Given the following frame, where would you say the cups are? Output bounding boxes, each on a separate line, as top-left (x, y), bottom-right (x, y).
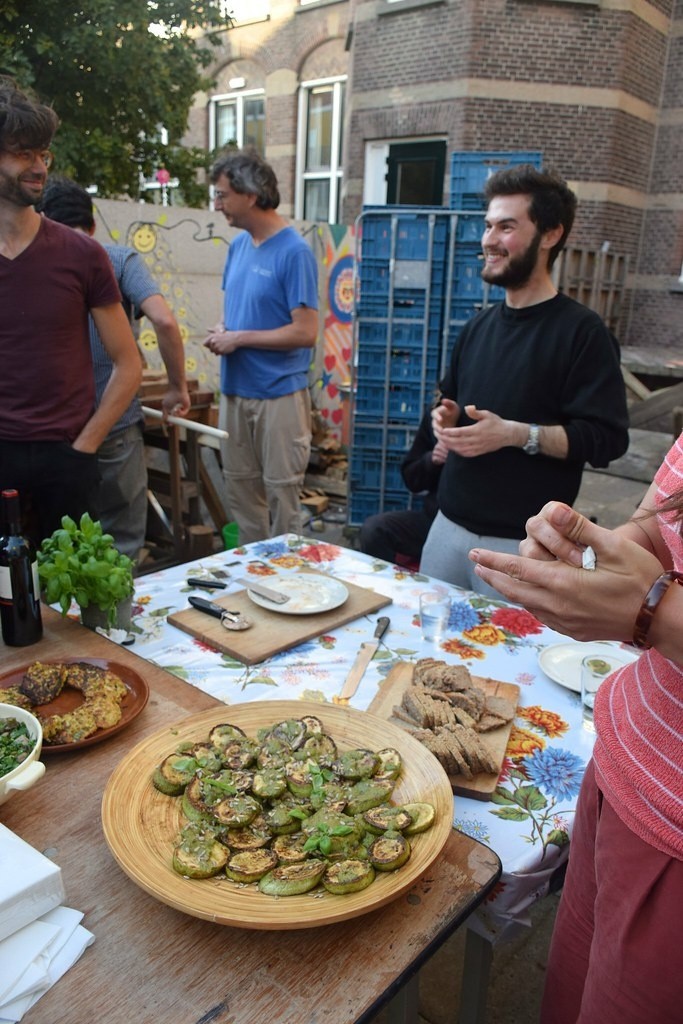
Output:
top-left (579, 653), bottom-right (627, 731)
top-left (418, 592), bottom-right (452, 642)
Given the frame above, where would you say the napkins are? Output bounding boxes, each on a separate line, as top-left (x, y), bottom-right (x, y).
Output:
top-left (0, 822), bottom-right (96, 1024)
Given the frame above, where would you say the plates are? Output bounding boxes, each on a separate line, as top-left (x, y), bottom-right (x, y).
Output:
top-left (0, 655), bottom-right (150, 751)
top-left (246, 571), bottom-right (349, 616)
top-left (537, 642), bottom-right (643, 696)
top-left (362, 661), bottom-right (520, 801)
top-left (100, 700), bottom-right (454, 930)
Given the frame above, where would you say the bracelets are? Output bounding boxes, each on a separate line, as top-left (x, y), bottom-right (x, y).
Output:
top-left (632, 569), bottom-right (683, 650)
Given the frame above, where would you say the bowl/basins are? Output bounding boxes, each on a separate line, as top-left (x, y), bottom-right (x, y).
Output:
top-left (0, 702), bottom-right (45, 812)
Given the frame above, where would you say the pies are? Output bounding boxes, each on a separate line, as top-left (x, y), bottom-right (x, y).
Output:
top-left (0, 660), bottom-right (126, 744)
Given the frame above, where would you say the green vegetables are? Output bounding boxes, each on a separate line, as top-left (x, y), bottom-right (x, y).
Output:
top-left (173, 727), bottom-right (352, 854)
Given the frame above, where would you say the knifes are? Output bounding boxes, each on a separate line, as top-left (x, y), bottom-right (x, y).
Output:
top-left (338, 616), bottom-right (390, 699)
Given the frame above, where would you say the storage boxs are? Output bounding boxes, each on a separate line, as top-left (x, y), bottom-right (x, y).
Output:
top-left (348, 152), bottom-right (542, 523)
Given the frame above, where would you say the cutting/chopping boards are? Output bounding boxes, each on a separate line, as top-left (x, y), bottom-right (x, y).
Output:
top-left (166, 566), bottom-right (393, 665)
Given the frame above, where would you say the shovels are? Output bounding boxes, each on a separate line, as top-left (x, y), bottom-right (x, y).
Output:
top-left (187, 578), bottom-right (290, 604)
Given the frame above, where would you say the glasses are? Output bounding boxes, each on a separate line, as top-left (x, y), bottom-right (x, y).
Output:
top-left (4, 150), bottom-right (53, 169)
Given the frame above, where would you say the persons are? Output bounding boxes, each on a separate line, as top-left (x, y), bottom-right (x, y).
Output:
top-left (418, 165), bottom-right (630, 608)
top-left (0, 72), bottom-right (144, 544)
top-left (467, 433), bottom-right (683, 1024)
top-left (32, 180), bottom-right (192, 579)
top-left (361, 388), bottom-right (448, 566)
top-left (204, 152), bottom-right (320, 544)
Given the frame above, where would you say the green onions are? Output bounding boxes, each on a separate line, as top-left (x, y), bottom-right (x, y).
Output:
top-left (0, 721), bottom-right (37, 776)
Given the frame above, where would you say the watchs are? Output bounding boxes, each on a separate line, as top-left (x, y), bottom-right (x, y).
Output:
top-left (522, 423), bottom-right (541, 455)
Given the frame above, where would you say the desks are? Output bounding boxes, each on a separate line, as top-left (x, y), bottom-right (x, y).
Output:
top-left (51, 532), bottom-right (645, 1024)
top-left (0, 603), bottom-right (501, 1024)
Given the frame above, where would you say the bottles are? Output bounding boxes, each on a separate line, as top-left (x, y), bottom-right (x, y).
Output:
top-left (0, 489), bottom-right (42, 648)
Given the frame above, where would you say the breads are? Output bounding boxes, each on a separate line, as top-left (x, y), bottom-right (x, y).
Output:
top-left (390, 658), bottom-right (516, 779)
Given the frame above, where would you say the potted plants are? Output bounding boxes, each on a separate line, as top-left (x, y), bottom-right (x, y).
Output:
top-left (36, 512), bottom-right (138, 631)
